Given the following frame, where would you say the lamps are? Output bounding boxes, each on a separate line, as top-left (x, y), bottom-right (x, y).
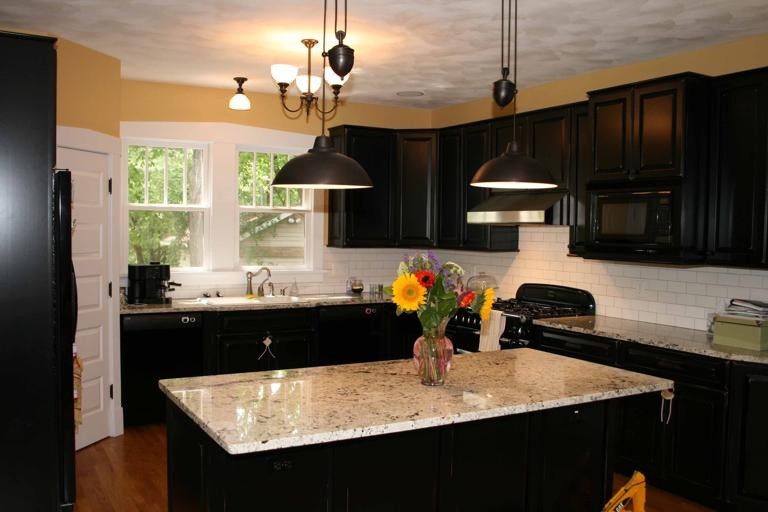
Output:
top-left (271, 40), bottom-right (351, 121)
top-left (229, 77), bottom-right (250, 111)
top-left (469, 1), bottom-right (558, 188)
top-left (271, 0), bottom-right (375, 189)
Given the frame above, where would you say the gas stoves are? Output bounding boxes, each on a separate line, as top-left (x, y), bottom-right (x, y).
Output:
top-left (492, 298), bottom-right (577, 320)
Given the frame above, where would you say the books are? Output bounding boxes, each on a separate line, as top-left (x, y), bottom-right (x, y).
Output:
top-left (725, 299), bottom-right (768, 317)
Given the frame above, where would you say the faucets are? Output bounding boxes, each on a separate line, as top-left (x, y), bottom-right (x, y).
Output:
top-left (245, 266), bottom-right (271, 296)
top-left (267, 282), bottom-right (276, 296)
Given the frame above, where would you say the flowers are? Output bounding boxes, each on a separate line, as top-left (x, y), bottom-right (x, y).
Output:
top-left (383, 251), bottom-right (494, 387)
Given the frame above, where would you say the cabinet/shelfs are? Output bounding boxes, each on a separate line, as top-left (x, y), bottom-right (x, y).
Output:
top-left (715, 67), bottom-right (768, 269)
top-left (588, 71), bottom-right (712, 182)
top-left (220, 308), bottom-right (317, 373)
top-left (308, 300), bottom-right (399, 368)
top-left (540, 327), bottom-right (617, 367)
top-left (396, 129), bottom-right (437, 249)
top-left (324, 124), bottom-right (396, 248)
top-left (618, 340), bottom-right (730, 512)
top-left (724, 362), bottom-right (768, 512)
top-left (491, 105), bottom-right (571, 251)
top-left (122, 311), bottom-right (212, 426)
top-left (439, 119), bottom-right (490, 253)
top-left (571, 100), bottom-right (594, 249)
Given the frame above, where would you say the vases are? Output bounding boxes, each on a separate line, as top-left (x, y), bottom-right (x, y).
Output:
top-left (412, 318), bottom-right (454, 386)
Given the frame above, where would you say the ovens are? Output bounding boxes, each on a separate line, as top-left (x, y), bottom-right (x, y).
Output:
top-left (455, 327), bottom-right (528, 356)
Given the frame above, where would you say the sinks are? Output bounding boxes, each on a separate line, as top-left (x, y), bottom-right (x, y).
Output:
top-left (201, 296), bottom-right (259, 306)
top-left (256, 296), bottom-right (298, 304)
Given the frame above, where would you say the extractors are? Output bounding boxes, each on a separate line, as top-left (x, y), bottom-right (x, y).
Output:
top-left (467, 192), bottom-right (570, 227)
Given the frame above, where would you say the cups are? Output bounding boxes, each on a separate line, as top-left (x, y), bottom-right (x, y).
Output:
top-left (370, 284), bottom-right (384, 293)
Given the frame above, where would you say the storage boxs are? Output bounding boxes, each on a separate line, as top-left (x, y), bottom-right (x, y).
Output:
top-left (712, 315), bottom-right (768, 351)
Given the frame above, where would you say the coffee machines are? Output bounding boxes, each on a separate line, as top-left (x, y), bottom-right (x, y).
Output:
top-left (127, 259), bottom-right (182, 304)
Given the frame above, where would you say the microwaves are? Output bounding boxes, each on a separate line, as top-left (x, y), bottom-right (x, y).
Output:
top-left (593, 191), bottom-right (672, 244)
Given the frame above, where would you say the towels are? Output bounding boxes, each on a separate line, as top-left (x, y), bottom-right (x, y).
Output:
top-left (477, 309), bottom-right (506, 352)
